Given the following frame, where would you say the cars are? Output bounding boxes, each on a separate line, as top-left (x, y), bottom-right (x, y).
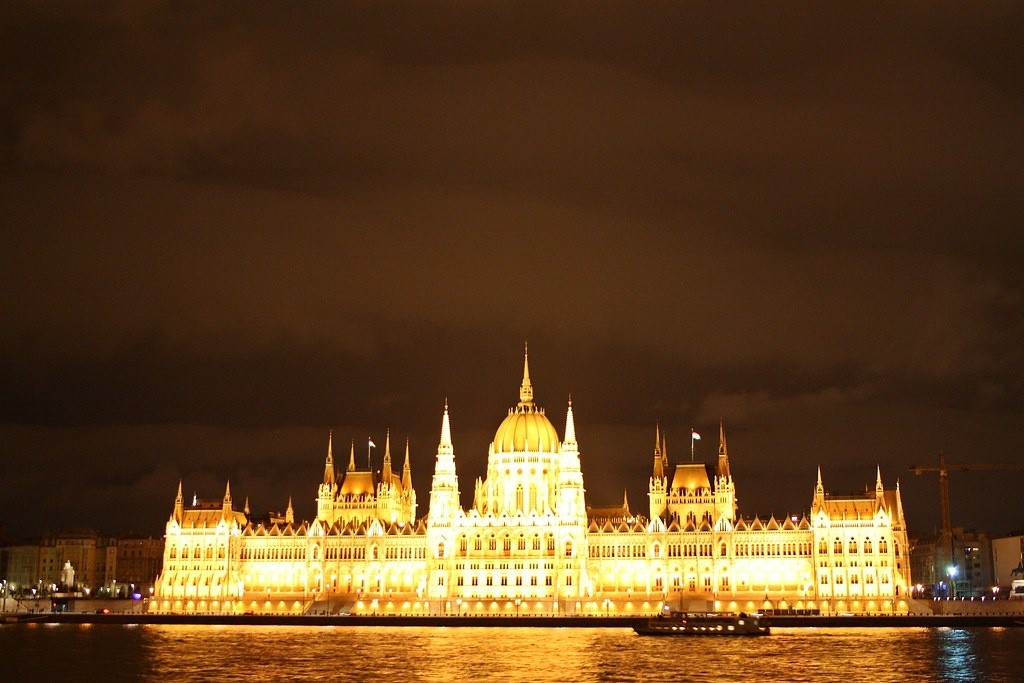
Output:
top-left (95, 609), bottom-right (109, 614)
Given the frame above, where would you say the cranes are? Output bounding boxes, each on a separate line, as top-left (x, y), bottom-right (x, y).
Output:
top-left (907, 450), bottom-right (1024, 577)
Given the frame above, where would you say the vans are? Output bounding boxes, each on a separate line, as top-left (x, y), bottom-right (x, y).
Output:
top-left (1008, 579), bottom-right (1024, 599)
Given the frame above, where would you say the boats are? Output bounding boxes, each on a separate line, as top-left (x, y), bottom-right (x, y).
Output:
top-left (627, 612), bottom-right (771, 636)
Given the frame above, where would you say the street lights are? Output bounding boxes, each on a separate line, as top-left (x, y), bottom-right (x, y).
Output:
top-left (605, 599), bottom-right (610, 617)
top-left (948, 567), bottom-right (957, 599)
top-left (514, 599), bottom-right (520, 617)
top-left (457, 599), bottom-right (462, 616)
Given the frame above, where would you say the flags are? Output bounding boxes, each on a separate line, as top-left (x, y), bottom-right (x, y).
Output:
top-left (369, 440), bottom-right (376, 447)
top-left (692, 432), bottom-right (701, 440)
